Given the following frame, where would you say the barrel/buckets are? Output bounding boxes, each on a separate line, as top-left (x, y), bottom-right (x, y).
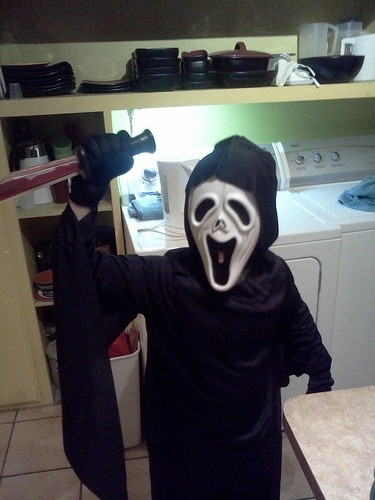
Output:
top-left (45, 340), bottom-right (62, 399)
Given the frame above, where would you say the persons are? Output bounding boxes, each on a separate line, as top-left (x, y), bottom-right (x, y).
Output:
top-left (50, 129), bottom-right (335, 500)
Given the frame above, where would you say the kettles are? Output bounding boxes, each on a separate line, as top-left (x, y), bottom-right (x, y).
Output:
top-left (156, 154), bottom-right (203, 234)
top-left (8, 136), bottom-right (56, 204)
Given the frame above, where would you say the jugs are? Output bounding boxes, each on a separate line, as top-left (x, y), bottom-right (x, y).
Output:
top-left (295, 22), bottom-right (337, 58)
top-left (339, 32), bottom-right (375, 82)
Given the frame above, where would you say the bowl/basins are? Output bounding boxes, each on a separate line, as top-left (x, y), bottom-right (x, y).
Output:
top-left (132, 40), bottom-right (278, 91)
top-left (297, 54), bottom-right (366, 85)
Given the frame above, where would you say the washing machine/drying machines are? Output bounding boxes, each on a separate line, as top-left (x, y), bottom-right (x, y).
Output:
top-left (254, 133), bottom-right (374, 401)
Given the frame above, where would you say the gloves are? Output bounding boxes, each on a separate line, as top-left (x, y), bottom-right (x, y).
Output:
top-left (70, 130), bottom-right (135, 206)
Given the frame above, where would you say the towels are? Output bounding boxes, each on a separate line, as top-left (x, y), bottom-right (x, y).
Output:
top-left (339, 175), bottom-right (375, 211)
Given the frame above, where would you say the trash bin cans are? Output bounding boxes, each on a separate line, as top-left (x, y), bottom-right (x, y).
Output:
top-left (109, 340), bottom-right (141, 449)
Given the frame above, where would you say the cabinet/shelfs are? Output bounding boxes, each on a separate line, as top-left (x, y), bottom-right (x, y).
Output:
top-left (0, 0), bottom-right (375, 412)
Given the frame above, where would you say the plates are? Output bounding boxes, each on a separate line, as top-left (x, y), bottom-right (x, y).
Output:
top-left (33, 269), bottom-right (54, 303)
top-left (80, 79), bottom-right (132, 93)
top-left (0, 60), bottom-right (77, 98)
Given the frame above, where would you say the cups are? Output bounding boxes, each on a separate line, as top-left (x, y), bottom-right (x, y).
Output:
top-left (335, 21), bottom-right (366, 54)
top-left (18, 191), bottom-right (34, 211)
top-left (50, 179), bottom-right (70, 203)
top-left (8, 83), bottom-right (24, 99)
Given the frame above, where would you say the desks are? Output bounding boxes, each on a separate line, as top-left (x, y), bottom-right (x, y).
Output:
top-left (283, 386), bottom-right (375, 500)
